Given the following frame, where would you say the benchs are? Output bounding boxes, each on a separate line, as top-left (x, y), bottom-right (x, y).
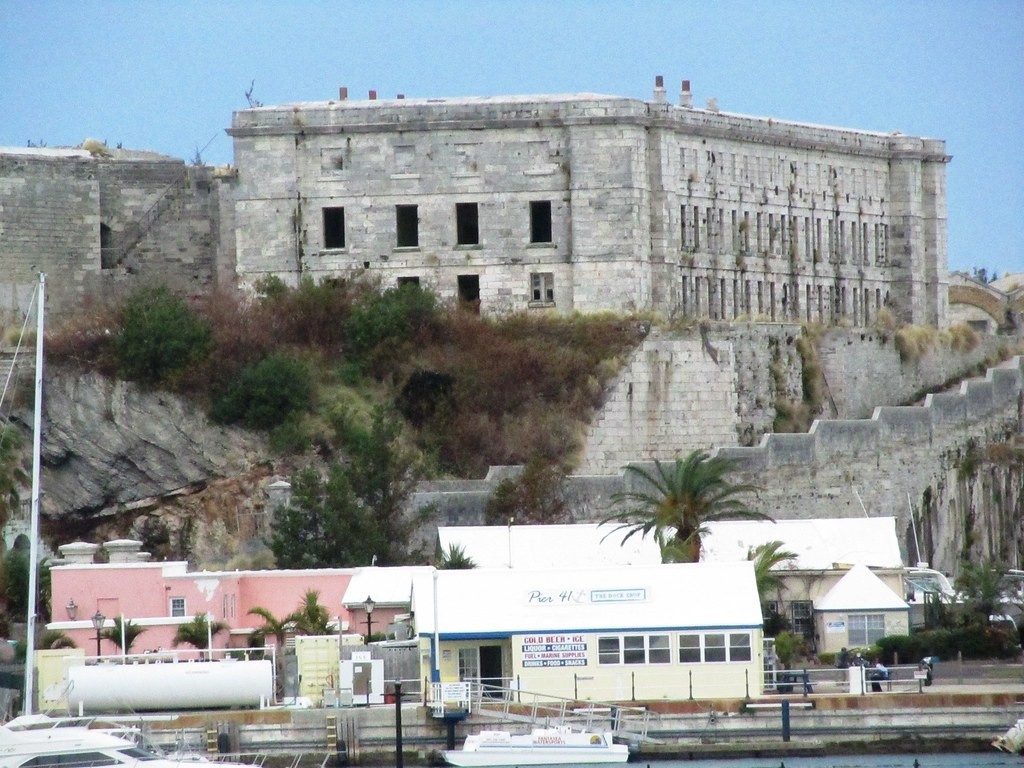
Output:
top-left (865, 671), bottom-right (892, 692)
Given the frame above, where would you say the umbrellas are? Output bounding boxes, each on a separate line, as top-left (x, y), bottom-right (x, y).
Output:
top-left (920, 657), bottom-right (938, 663)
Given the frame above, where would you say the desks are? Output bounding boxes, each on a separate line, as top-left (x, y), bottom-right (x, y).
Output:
top-left (782, 674), bottom-right (813, 693)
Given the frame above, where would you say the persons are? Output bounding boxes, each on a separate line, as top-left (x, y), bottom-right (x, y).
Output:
top-left (922, 663), bottom-right (934, 686)
top-left (871, 658), bottom-right (888, 692)
top-left (835, 647), bottom-right (850, 686)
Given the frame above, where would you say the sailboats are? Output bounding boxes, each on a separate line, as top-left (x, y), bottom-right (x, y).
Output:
top-left (0, 270), bottom-right (351, 768)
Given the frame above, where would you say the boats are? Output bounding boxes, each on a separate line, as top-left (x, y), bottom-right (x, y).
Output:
top-left (852, 488), bottom-right (1024, 648)
top-left (437, 715), bottom-right (633, 768)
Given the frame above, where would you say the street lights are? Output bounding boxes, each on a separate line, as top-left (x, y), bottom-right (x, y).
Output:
top-left (88, 610), bottom-right (110, 656)
top-left (359, 595), bottom-right (379, 643)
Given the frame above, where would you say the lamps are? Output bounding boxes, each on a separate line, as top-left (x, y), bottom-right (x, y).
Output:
top-left (65, 598), bottom-right (78, 621)
